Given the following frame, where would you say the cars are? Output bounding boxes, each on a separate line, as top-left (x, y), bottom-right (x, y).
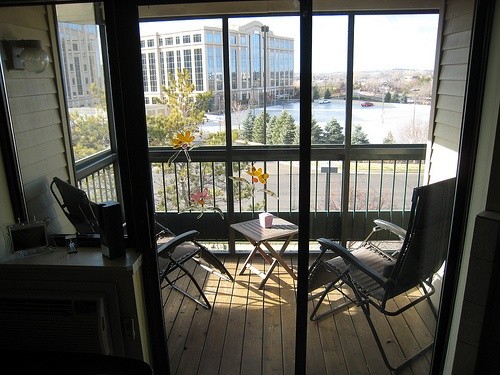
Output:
top-left (318, 98), bottom-right (331, 104)
top-left (361, 102), bottom-right (373, 107)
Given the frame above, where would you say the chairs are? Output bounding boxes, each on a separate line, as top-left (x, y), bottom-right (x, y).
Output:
top-left (49, 176), bottom-right (235, 310)
top-left (296, 175), bottom-right (457, 372)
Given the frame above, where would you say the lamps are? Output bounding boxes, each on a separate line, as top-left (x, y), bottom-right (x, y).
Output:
top-left (2, 39), bottom-right (50, 74)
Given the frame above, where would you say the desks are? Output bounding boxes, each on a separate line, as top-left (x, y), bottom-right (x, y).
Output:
top-left (229, 214), bottom-right (299, 292)
